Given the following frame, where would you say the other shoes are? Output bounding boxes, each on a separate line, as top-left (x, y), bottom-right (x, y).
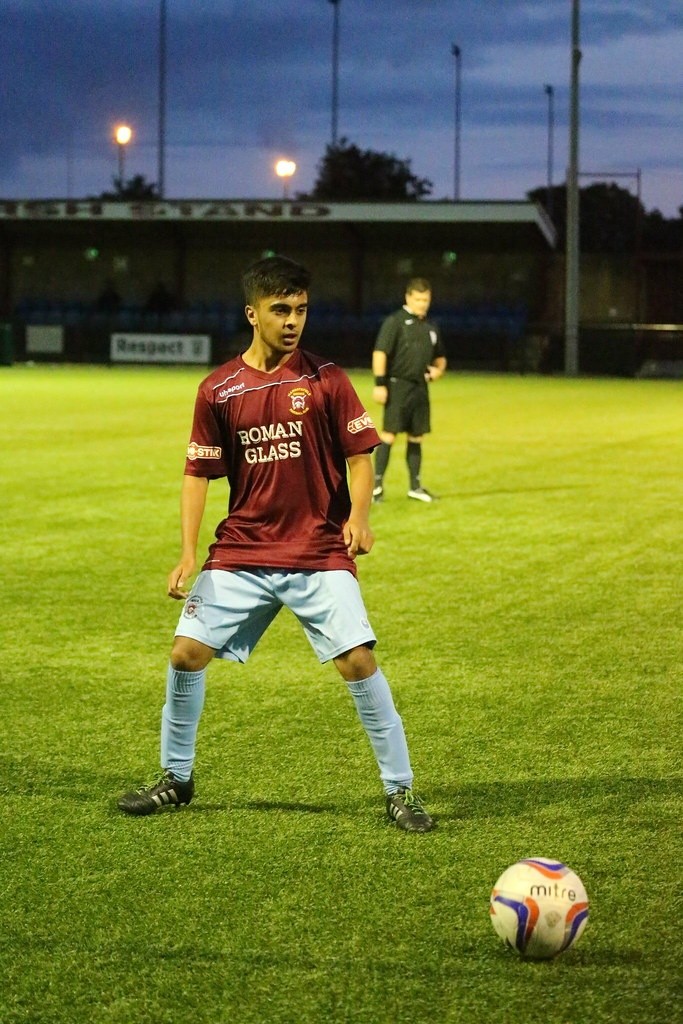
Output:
top-left (372, 485), bottom-right (384, 502)
top-left (407, 487), bottom-right (434, 503)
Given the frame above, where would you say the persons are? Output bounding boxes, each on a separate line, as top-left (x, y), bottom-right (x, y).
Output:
top-left (117, 256), bottom-right (434, 833)
top-left (97, 287), bottom-right (121, 313)
top-left (140, 283), bottom-right (174, 314)
top-left (370, 277), bottom-right (447, 502)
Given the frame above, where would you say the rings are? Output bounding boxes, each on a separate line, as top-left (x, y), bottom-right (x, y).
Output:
top-left (430, 378), bottom-right (432, 380)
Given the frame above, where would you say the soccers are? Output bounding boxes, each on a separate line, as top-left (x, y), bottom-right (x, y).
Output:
top-left (488, 856), bottom-right (590, 959)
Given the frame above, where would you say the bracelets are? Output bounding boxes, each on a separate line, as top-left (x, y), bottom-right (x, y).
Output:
top-left (375, 377), bottom-right (386, 386)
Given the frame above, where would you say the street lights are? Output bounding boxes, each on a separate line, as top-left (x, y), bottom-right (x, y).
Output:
top-left (543, 83), bottom-right (554, 187)
top-left (116, 126), bottom-right (131, 192)
top-left (449, 44), bottom-right (463, 202)
top-left (275, 159), bottom-right (298, 201)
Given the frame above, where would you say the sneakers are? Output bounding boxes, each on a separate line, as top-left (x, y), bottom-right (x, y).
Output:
top-left (385, 785), bottom-right (433, 833)
top-left (117, 771), bottom-right (195, 815)
